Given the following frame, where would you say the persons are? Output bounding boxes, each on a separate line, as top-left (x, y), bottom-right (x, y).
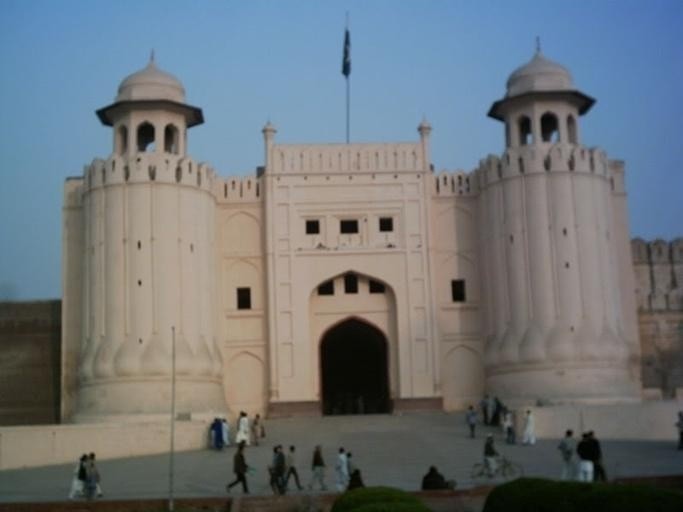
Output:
top-left (207, 411), bottom-right (262, 447)
top-left (556, 428), bottom-right (606, 483)
top-left (674, 410), bottom-right (682, 451)
top-left (226, 443), bottom-right (249, 494)
top-left (69, 453), bottom-right (103, 500)
top-left (483, 434), bottom-right (499, 479)
top-left (421, 466), bottom-right (447, 489)
top-left (268, 445), bottom-right (363, 494)
top-left (464, 394), bottom-right (536, 446)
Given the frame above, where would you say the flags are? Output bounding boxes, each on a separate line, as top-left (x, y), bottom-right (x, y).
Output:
top-left (341, 30), bottom-right (351, 76)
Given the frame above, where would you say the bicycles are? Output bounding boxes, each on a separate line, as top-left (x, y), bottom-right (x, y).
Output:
top-left (472, 449), bottom-right (523, 486)
